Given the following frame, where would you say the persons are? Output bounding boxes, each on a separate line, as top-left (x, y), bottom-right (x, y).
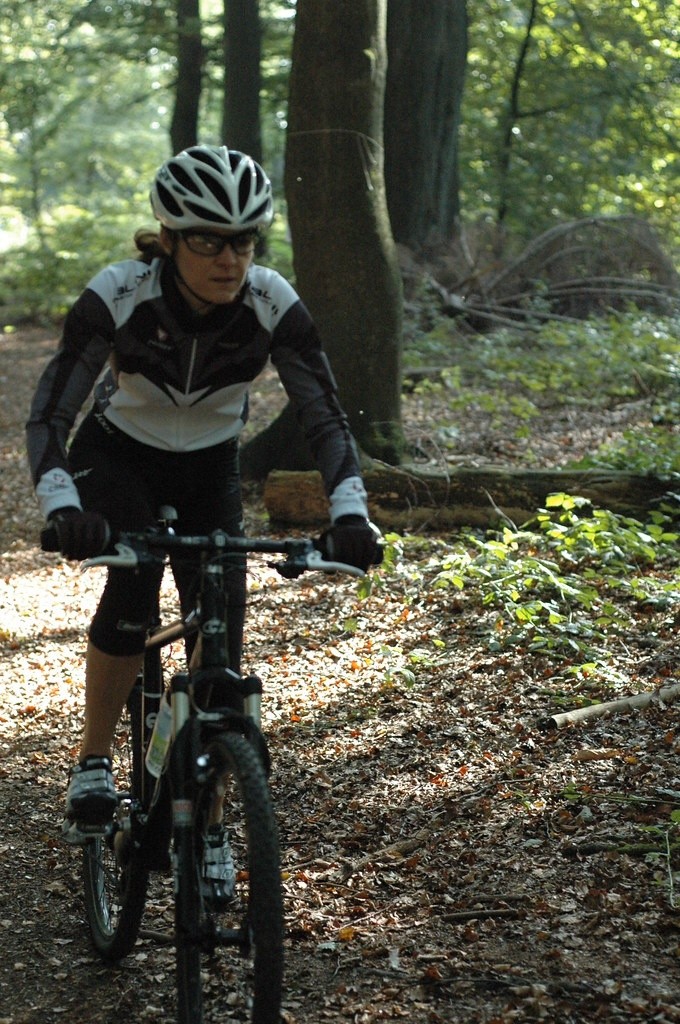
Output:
top-left (26, 144), bottom-right (383, 915)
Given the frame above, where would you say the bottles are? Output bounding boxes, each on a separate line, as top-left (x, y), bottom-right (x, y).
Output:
top-left (145, 687), bottom-right (171, 778)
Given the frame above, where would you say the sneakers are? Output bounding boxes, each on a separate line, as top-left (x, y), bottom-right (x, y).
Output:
top-left (66, 754), bottom-right (118, 821)
top-left (203, 823), bottom-right (236, 902)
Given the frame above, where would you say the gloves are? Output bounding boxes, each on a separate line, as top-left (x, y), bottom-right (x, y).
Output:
top-left (318, 515), bottom-right (383, 574)
top-left (49, 509), bottom-right (117, 561)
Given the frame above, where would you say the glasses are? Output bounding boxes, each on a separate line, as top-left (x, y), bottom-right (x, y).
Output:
top-left (180, 227), bottom-right (263, 255)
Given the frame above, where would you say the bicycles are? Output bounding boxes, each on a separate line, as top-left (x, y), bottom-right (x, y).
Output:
top-left (37, 504), bottom-right (387, 1023)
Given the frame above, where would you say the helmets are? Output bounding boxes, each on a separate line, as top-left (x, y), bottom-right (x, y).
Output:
top-left (150, 143), bottom-right (273, 233)
